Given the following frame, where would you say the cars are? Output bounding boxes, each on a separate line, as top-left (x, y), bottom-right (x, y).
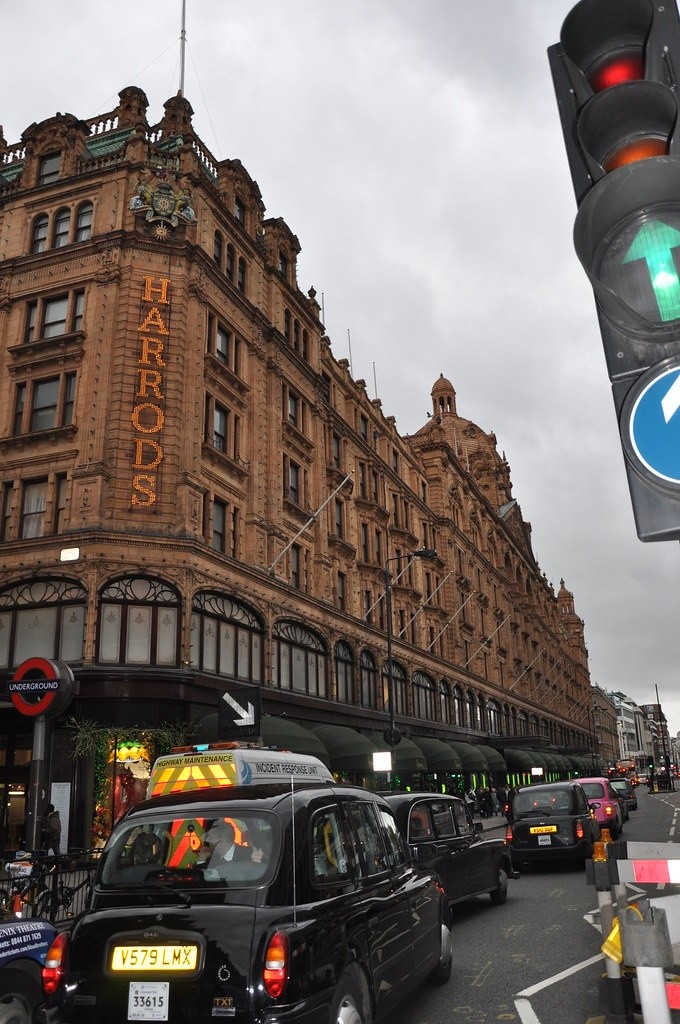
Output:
top-left (40, 741), bottom-right (453, 1024)
top-left (381, 792), bottom-right (511, 905)
top-left (571, 777), bottom-right (625, 841)
top-left (505, 780), bottom-right (602, 880)
top-left (611, 758), bottom-right (651, 824)
top-left (0, 916), bottom-right (59, 1024)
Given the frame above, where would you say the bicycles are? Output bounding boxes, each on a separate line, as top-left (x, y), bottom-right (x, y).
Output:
top-left (0, 849), bottom-right (104, 922)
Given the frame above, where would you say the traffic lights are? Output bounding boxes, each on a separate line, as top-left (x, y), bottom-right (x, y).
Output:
top-left (647, 756), bottom-right (654, 770)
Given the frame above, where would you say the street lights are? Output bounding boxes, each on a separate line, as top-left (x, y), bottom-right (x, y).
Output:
top-left (592, 707), bottom-right (609, 776)
top-left (384, 547), bottom-right (438, 791)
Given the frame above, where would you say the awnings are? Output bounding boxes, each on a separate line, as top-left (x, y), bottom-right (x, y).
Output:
top-left (261, 714), bottom-right (610, 769)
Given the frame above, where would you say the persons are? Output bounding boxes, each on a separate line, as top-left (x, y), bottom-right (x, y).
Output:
top-left (416, 784), bottom-right (516, 820)
top-left (204, 822), bottom-right (278, 869)
top-left (40, 805), bottom-right (76, 871)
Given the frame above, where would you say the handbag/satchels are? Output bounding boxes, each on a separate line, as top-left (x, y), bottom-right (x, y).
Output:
top-left (496, 805), bottom-right (501, 812)
top-left (468, 792), bottom-right (478, 802)
